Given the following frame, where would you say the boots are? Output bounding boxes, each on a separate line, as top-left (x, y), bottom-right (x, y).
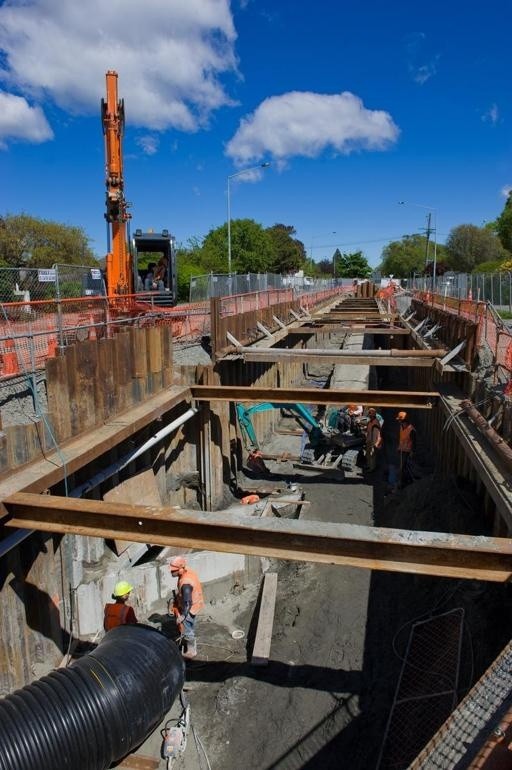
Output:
top-left (183, 639), bottom-right (196, 658)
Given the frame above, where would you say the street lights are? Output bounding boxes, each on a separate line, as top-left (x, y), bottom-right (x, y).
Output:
top-left (398, 200), bottom-right (437, 290)
top-left (310, 231), bottom-right (336, 273)
top-left (226, 162), bottom-right (270, 276)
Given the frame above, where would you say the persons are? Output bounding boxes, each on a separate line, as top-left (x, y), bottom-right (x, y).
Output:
top-left (167, 554), bottom-right (204, 659)
top-left (102, 581), bottom-right (138, 631)
top-left (143, 258), bottom-right (169, 292)
top-left (339, 405), bottom-right (419, 494)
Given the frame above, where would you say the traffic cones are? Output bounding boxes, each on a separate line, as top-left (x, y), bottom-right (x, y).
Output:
top-left (88, 313), bottom-right (97, 340)
top-left (3, 335), bottom-right (18, 374)
top-left (47, 321), bottom-right (58, 358)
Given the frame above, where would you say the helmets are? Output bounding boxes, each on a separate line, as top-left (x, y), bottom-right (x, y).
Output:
top-left (396, 412), bottom-right (408, 419)
top-left (368, 408), bottom-right (376, 416)
top-left (170, 557), bottom-right (186, 570)
top-left (114, 581), bottom-right (134, 597)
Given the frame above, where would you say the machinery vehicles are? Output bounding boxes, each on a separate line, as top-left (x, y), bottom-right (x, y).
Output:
top-left (103, 68), bottom-right (182, 314)
top-left (234, 402), bottom-right (386, 478)
